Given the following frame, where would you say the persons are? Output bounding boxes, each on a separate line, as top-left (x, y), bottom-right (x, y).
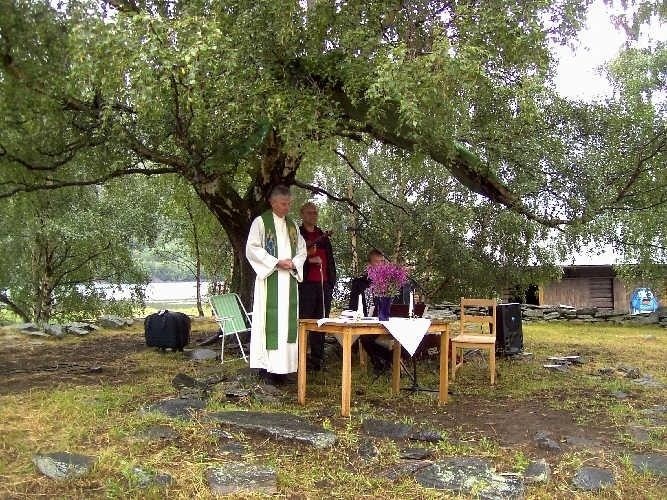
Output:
top-left (245, 186), bottom-right (308, 379)
top-left (298, 202), bottom-right (337, 371)
top-left (348, 248), bottom-right (394, 374)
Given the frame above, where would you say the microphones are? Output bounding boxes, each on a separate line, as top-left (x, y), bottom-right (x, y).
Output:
top-left (345, 226), bottom-right (364, 232)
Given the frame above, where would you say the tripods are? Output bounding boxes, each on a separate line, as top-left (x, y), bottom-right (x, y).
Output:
top-left (400, 290), bottom-right (452, 398)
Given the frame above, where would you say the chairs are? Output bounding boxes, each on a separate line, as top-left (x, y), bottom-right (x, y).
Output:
top-left (206, 291), bottom-right (252, 365)
top-left (450, 295), bottom-right (499, 386)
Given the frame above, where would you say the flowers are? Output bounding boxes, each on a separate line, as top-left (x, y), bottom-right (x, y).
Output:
top-left (365, 261), bottom-right (410, 300)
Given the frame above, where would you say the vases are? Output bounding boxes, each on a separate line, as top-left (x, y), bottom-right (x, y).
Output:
top-left (372, 296), bottom-right (394, 321)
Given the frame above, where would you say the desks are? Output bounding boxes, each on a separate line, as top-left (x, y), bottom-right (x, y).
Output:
top-left (294, 317), bottom-right (454, 418)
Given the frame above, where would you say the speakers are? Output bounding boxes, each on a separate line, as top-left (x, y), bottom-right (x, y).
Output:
top-left (489, 302), bottom-right (522, 355)
